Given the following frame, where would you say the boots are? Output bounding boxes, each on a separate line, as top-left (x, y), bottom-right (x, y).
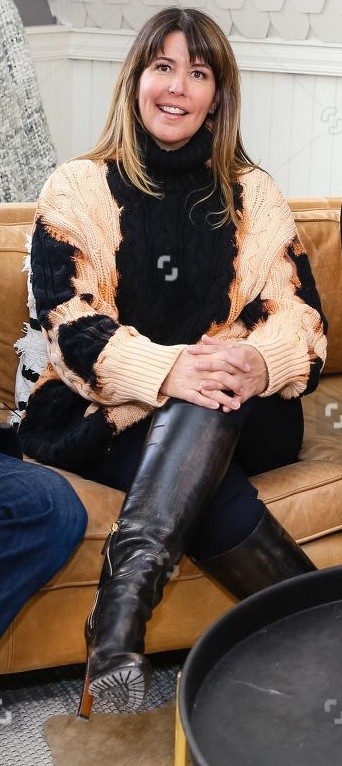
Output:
top-left (77, 399), bottom-right (243, 718)
top-left (190, 498), bottom-right (318, 602)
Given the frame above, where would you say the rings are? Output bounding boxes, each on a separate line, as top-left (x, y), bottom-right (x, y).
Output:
top-left (199, 380), bottom-right (205, 390)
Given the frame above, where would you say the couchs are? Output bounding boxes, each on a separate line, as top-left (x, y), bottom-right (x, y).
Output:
top-left (0, 195), bottom-right (342, 679)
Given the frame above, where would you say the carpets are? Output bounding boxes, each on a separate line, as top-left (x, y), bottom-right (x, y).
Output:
top-left (43, 697), bottom-right (175, 766)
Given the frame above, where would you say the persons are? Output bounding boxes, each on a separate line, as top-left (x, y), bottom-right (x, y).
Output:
top-left (12, 6), bottom-right (328, 717)
top-left (0, 423), bottom-right (90, 703)
